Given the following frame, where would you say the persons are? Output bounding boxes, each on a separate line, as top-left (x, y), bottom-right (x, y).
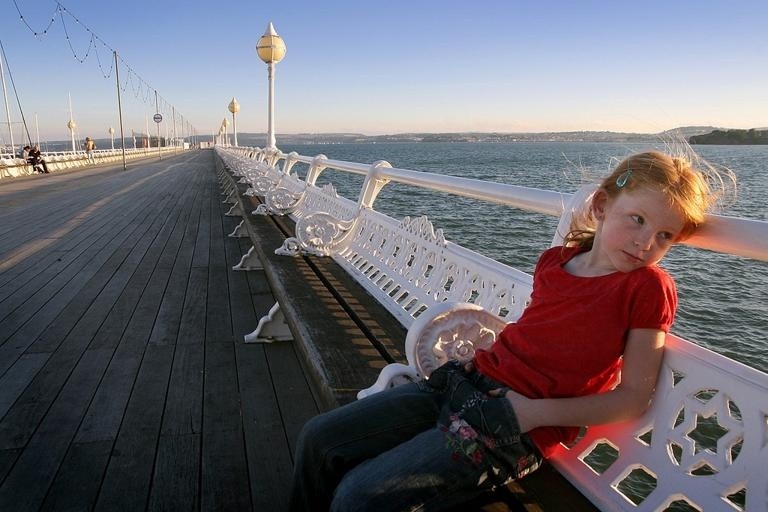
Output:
top-left (84, 137), bottom-right (96, 163)
top-left (287, 151), bottom-right (710, 512)
top-left (21, 146), bottom-right (39, 174)
top-left (28, 144), bottom-right (50, 174)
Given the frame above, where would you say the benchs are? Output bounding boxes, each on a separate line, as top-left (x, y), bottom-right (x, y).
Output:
top-left (213, 146), bottom-right (767, 512)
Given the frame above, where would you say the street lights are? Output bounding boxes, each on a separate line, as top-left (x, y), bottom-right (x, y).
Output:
top-left (216, 119), bottom-right (228, 145)
top-left (67, 120), bottom-right (78, 152)
top-left (108, 128), bottom-right (115, 150)
top-left (228, 98), bottom-right (240, 144)
top-left (257, 22), bottom-right (287, 146)
top-left (131, 127), bottom-right (136, 148)
